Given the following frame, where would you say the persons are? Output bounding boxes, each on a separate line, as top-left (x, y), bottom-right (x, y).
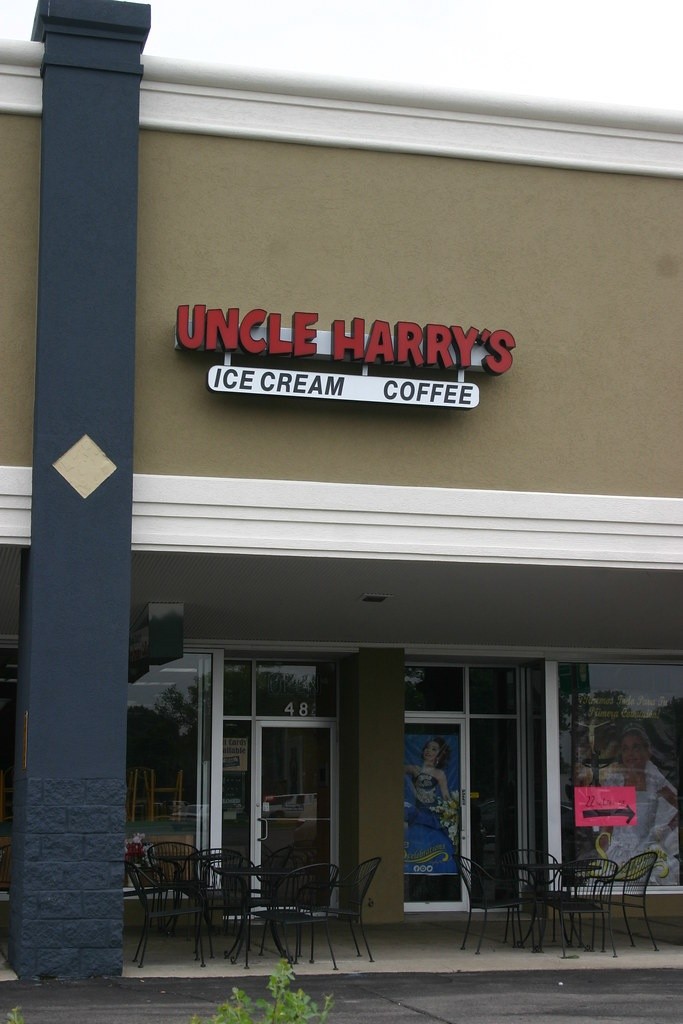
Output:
top-left (575, 721), bottom-right (679, 886)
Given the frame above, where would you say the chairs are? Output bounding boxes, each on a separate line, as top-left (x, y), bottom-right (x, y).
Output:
top-left (450, 848), bottom-right (660, 958)
top-left (126, 840), bottom-right (383, 975)
top-left (125, 767), bottom-right (184, 821)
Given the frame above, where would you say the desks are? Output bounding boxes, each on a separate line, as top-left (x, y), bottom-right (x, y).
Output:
top-left (214, 867), bottom-right (304, 966)
top-left (510, 862), bottom-right (605, 946)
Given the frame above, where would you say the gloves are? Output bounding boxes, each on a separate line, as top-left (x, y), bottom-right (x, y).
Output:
top-left (648, 823), bottom-right (672, 844)
